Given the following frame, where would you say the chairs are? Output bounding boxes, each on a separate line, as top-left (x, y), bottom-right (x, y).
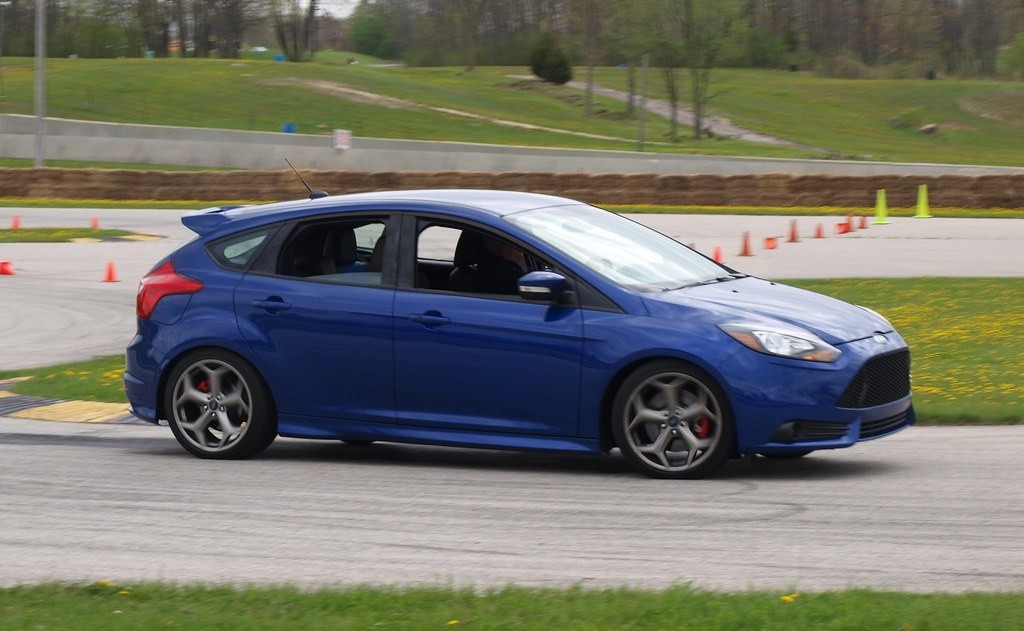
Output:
top-left (281, 220), bottom-right (480, 292)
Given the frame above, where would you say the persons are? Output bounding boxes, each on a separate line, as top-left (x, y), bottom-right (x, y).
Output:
top-left (471, 234), bottom-right (526, 293)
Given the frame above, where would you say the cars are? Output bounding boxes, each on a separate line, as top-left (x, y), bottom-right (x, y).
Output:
top-left (121, 156), bottom-right (920, 480)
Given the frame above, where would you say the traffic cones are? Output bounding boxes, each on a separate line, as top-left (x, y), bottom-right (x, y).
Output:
top-left (0, 262), bottom-right (15, 276)
top-left (873, 189), bottom-right (890, 226)
top-left (713, 210), bottom-right (869, 264)
top-left (100, 261), bottom-right (120, 283)
top-left (913, 182), bottom-right (934, 219)
top-left (7, 214), bottom-right (100, 230)
